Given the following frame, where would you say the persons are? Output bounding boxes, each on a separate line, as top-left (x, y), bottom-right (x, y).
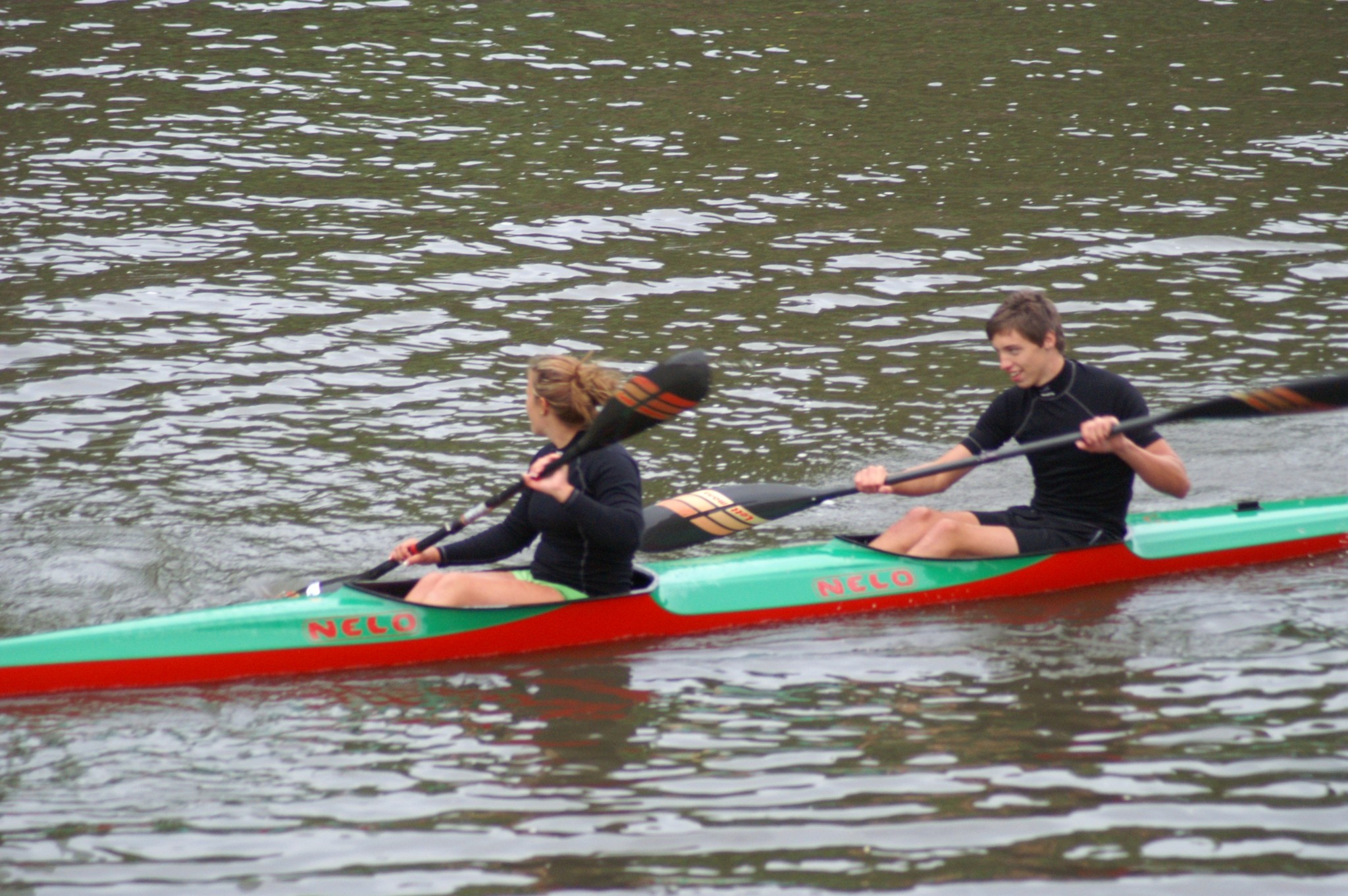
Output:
top-left (854, 293), bottom-right (1190, 559)
top-left (403, 351), bottom-right (645, 608)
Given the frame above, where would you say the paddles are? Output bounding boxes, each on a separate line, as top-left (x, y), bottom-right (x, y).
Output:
top-left (298, 348), bottom-right (715, 600)
top-left (631, 375), bottom-right (1348, 554)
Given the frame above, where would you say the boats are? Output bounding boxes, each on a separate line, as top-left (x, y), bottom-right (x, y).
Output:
top-left (0, 493), bottom-right (1346, 699)
